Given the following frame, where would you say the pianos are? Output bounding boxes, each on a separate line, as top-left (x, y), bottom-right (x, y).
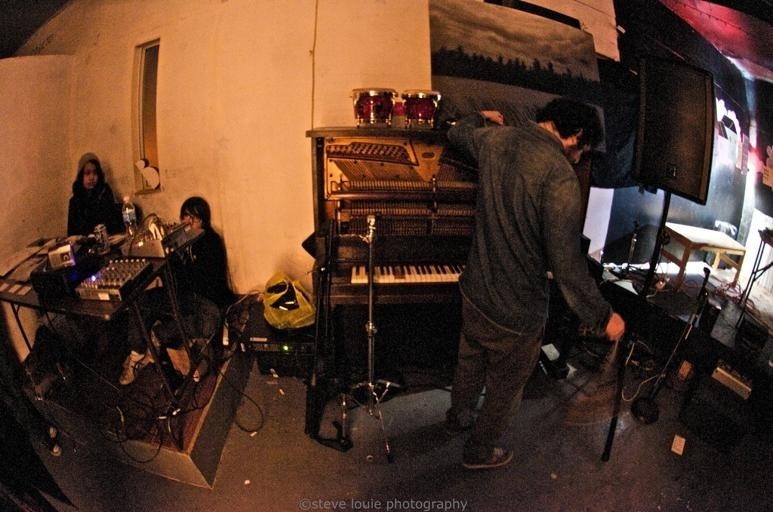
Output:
top-left (305, 126), bottom-right (604, 440)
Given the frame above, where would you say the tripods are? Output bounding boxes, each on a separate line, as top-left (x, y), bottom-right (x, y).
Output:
top-left (571, 192), bottom-right (674, 462)
top-left (343, 244), bottom-right (402, 465)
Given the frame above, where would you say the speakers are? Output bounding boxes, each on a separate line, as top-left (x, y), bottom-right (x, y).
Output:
top-left (632, 50), bottom-right (720, 205)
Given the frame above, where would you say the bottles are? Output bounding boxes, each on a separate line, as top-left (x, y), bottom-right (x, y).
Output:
top-left (122, 196), bottom-right (139, 240)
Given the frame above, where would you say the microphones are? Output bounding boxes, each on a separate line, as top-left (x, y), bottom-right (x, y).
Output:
top-left (364, 213), bottom-right (376, 241)
top-left (431, 174), bottom-right (439, 213)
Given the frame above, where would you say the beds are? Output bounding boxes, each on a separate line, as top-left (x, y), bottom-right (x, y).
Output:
top-left (661, 223), bottom-right (746, 290)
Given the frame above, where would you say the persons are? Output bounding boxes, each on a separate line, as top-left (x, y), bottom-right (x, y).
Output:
top-left (444, 97), bottom-right (627, 472)
top-left (0, 329), bottom-right (62, 457)
top-left (117, 194), bottom-right (231, 388)
top-left (65, 151), bottom-right (124, 329)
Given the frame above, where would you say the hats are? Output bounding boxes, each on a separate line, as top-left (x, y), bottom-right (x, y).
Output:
top-left (78, 153), bottom-right (100, 174)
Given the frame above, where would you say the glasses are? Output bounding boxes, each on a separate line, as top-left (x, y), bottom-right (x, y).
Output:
top-left (183, 214), bottom-right (200, 223)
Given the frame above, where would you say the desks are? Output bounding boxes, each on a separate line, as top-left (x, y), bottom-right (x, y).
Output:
top-left (0, 258), bottom-right (190, 402)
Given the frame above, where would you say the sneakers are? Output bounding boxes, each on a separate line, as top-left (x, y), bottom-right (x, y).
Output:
top-left (464, 446), bottom-right (513, 469)
top-left (119, 320), bottom-right (162, 384)
top-left (446, 407), bottom-right (479, 433)
